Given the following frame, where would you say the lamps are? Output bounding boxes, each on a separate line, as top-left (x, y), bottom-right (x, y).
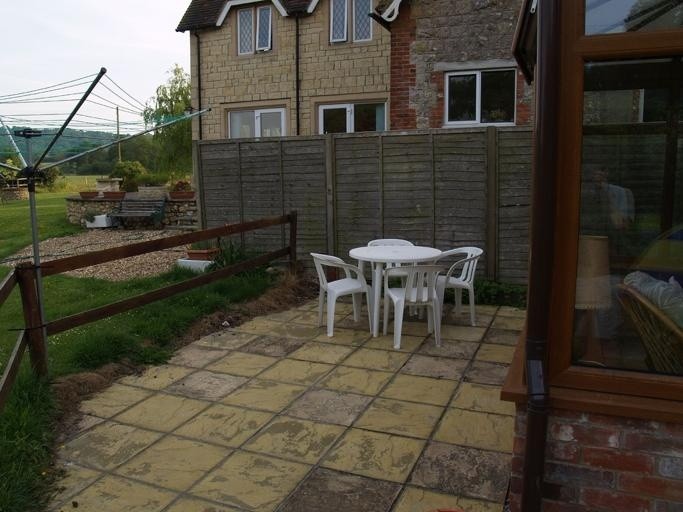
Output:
top-left (575, 235), bottom-right (612, 366)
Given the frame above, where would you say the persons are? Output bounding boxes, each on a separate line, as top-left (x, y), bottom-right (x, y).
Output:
top-left (573, 141), bottom-right (636, 367)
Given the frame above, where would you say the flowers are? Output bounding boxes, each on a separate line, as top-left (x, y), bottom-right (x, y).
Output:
top-left (166, 171), bottom-right (192, 192)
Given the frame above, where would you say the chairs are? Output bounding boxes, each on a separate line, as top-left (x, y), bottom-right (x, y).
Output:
top-left (614, 284), bottom-right (683, 374)
top-left (310, 238), bottom-right (483, 350)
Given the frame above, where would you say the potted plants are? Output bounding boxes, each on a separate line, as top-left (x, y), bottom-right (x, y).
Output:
top-left (81, 209), bottom-right (118, 228)
top-left (186, 238), bottom-right (225, 260)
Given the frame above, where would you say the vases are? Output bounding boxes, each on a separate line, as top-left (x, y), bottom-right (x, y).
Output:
top-left (177, 258), bottom-right (215, 273)
top-left (103, 191), bottom-right (126, 198)
top-left (170, 192), bottom-right (195, 199)
top-left (80, 192), bottom-right (97, 198)
